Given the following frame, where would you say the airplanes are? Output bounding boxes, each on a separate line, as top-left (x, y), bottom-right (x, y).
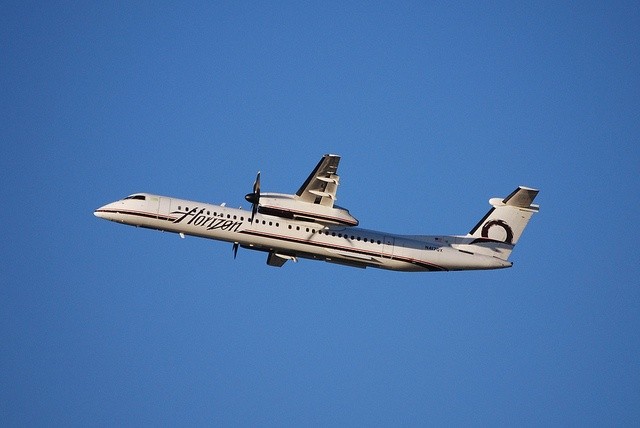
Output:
top-left (93, 154), bottom-right (540, 272)
